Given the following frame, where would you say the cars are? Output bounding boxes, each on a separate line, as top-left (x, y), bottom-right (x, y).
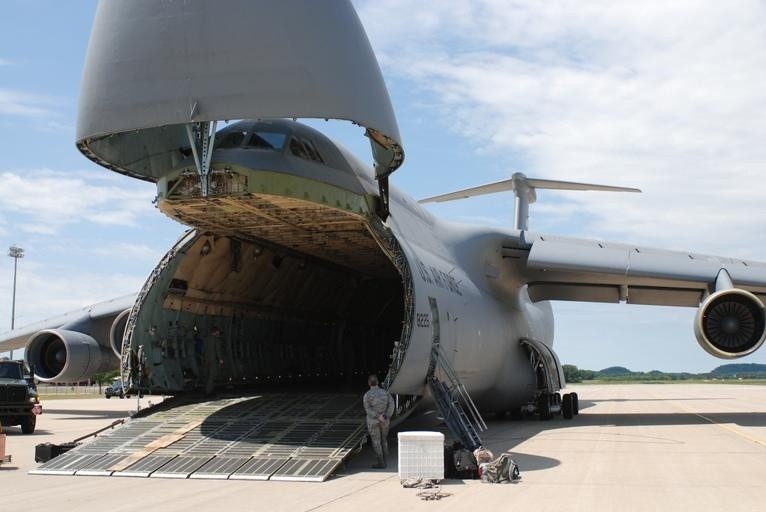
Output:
top-left (105, 380), bottom-right (144, 399)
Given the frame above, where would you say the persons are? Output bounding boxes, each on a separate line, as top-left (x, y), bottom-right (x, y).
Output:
top-left (205, 326), bottom-right (225, 397)
top-left (363, 375), bottom-right (395, 468)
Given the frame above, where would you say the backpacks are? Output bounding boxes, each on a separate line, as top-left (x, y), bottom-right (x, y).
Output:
top-left (448, 442), bottom-right (519, 483)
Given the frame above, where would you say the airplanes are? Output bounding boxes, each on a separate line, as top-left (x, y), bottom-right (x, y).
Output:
top-left (0, 0), bottom-right (766, 482)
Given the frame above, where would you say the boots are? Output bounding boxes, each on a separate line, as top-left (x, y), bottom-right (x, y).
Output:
top-left (372, 455), bottom-right (387, 468)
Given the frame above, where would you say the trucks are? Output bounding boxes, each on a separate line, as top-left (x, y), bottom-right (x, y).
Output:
top-left (0, 357), bottom-right (42, 433)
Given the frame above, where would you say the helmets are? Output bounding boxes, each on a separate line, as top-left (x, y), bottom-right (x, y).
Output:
top-left (368, 375), bottom-right (378, 385)
